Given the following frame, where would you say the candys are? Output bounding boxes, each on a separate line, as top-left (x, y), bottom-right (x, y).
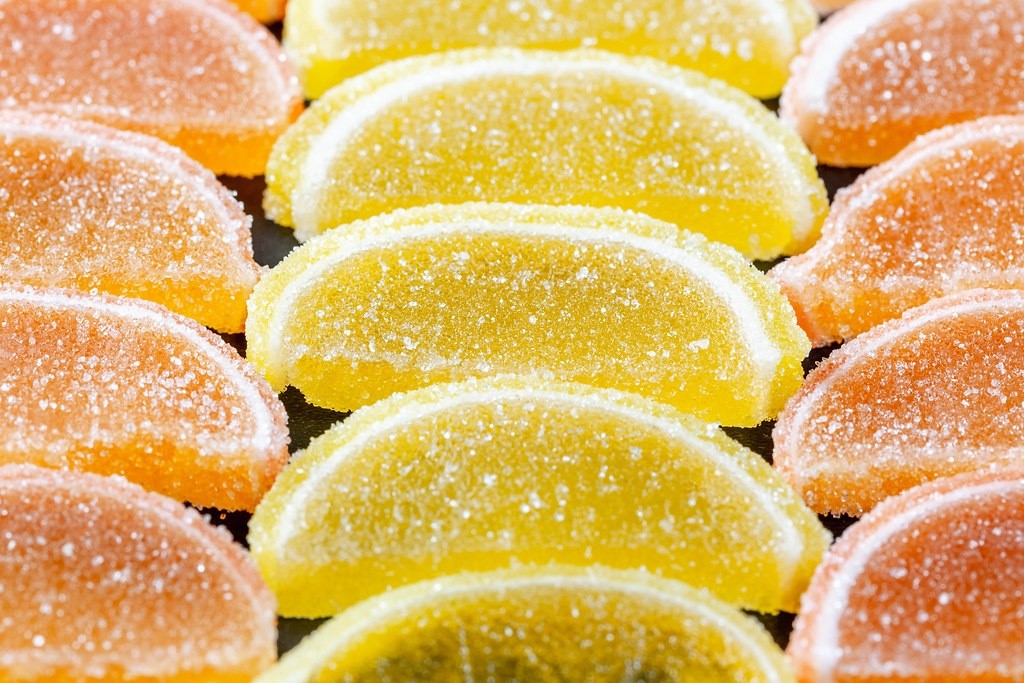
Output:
top-left (0, 0), bottom-right (1024, 683)
